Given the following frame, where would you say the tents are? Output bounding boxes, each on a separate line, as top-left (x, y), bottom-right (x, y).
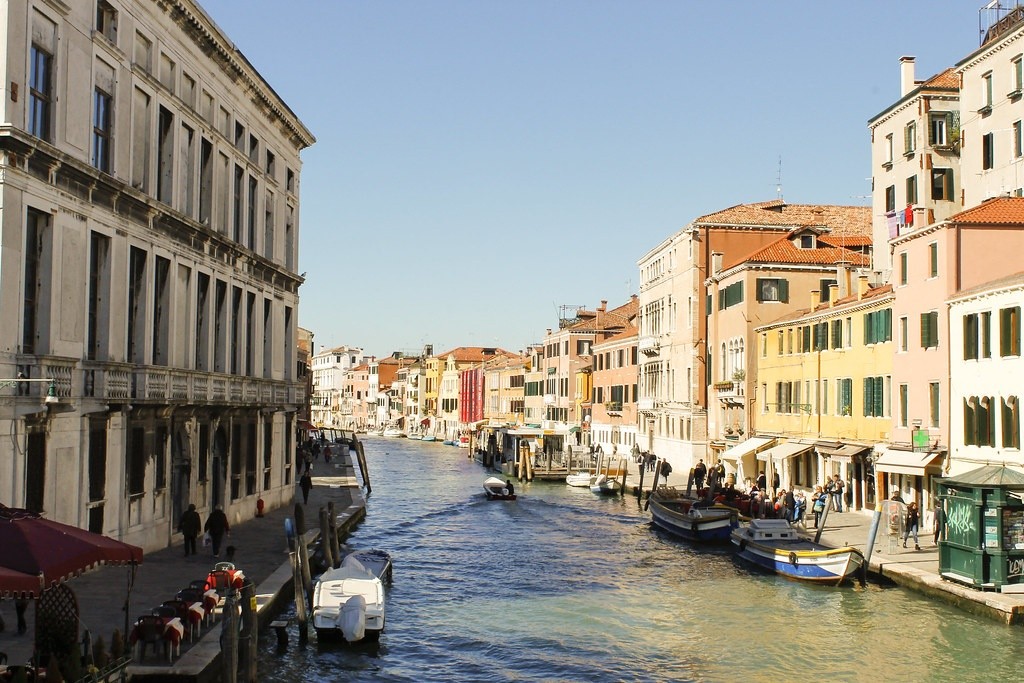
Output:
top-left (0, 502), bottom-right (142, 648)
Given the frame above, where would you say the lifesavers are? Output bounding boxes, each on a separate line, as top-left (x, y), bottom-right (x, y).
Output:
top-left (691, 523), bottom-right (698, 537)
top-left (788, 552), bottom-right (796, 565)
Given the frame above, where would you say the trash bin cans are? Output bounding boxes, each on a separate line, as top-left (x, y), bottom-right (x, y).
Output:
top-left (727, 473), bottom-right (734, 485)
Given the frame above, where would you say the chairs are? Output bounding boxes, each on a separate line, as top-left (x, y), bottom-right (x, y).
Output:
top-left (137, 561), bottom-right (236, 664)
top-left (0, 652), bottom-right (8, 665)
top-left (6, 665), bottom-right (35, 683)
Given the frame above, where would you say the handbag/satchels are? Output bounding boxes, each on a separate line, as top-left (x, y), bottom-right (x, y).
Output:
top-left (813, 504), bottom-right (822, 512)
top-left (202, 530), bottom-right (211, 547)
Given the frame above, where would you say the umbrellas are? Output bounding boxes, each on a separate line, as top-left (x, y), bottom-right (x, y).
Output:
top-left (735, 458), bottom-right (746, 494)
top-left (764, 453), bottom-right (774, 497)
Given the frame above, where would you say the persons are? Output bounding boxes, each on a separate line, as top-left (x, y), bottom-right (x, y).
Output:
top-left (720, 469), bottom-right (806, 522)
top-left (13, 593), bottom-right (29, 637)
top-left (660, 458), bottom-right (672, 483)
top-left (812, 474), bottom-right (844, 527)
top-left (589, 442), bottom-right (602, 461)
top-left (296, 432), bottom-right (331, 474)
top-left (931, 504), bottom-right (947, 545)
top-left (630, 443), bottom-right (656, 473)
top-left (505, 480), bottom-right (514, 495)
top-left (204, 504), bottom-right (230, 557)
top-left (891, 491), bottom-right (904, 503)
top-left (177, 503), bottom-right (201, 557)
top-left (902, 501), bottom-right (920, 550)
top-left (299, 471), bottom-right (313, 505)
top-left (693, 458), bottom-right (725, 490)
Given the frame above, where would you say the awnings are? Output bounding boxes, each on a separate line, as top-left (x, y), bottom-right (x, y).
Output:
top-left (831, 444), bottom-right (936, 476)
top-left (419, 417), bottom-right (428, 424)
top-left (720, 437), bottom-right (814, 461)
top-left (299, 421), bottom-right (318, 432)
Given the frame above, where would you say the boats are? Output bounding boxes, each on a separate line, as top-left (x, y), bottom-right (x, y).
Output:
top-left (483, 477), bottom-right (517, 500)
top-left (566, 472), bottom-right (621, 493)
top-left (335, 437), bottom-right (353, 444)
top-left (649, 490), bottom-right (740, 543)
top-left (443, 440), bottom-right (469, 447)
top-left (313, 547), bottom-right (392, 642)
top-left (729, 518), bottom-right (865, 587)
top-left (383, 429), bottom-right (436, 441)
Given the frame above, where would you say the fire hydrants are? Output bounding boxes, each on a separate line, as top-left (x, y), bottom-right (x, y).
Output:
top-left (256, 499), bottom-right (265, 518)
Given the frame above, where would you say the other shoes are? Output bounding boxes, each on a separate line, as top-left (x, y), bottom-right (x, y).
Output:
top-left (904, 541), bottom-right (907, 547)
top-left (213, 554), bottom-right (218, 557)
top-left (185, 552), bottom-right (189, 557)
top-left (931, 540), bottom-right (937, 545)
top-left (915, 544), bottom-right (920, 550)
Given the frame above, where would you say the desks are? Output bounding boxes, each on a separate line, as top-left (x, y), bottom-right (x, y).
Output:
top-left (159, 603), bottom-right (204, 640)
top-left (176, 590), bottom-right (219, 628)
top-left (205, 570), bottom-right (245, 601)
top-left (134, 617), bottom-right (183, 665)
top-left (0, 665), bottom-right (46, 683)
